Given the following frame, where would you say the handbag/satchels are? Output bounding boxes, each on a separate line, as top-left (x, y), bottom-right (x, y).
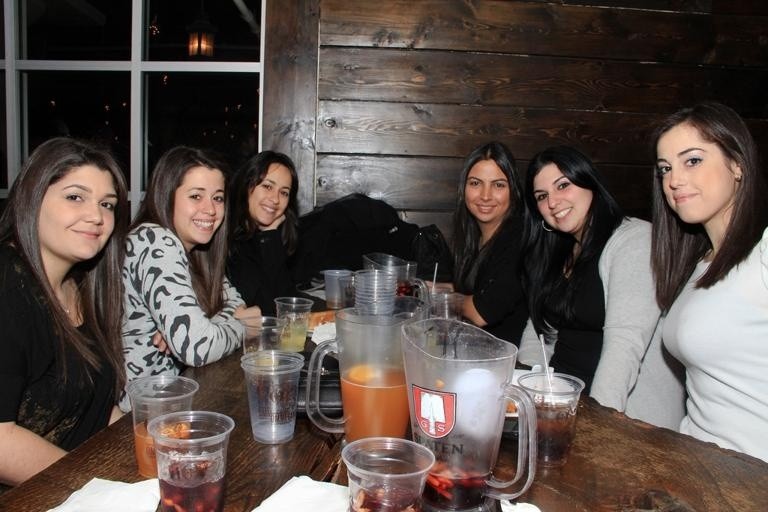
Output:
top-left (410, 223), bottom-right (455, 282)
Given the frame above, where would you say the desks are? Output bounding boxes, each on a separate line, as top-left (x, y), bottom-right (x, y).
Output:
top-left (0, 346), bottom-right (768, 512)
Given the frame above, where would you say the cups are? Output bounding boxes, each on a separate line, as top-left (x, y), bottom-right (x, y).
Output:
top-left (149, 410), bottom-right (235, 512)
top-left (125, 372), bottom-right (199, 478)
top-left (238, 254), bottom-right (588, 512)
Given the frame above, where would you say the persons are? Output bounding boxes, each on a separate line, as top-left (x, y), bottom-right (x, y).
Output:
top-left (0, 137), bottom-right (128, 489)
top-left (647, 100), bottom-right (768, 466)
top-left (420, 140), bottom-right (530, 359)
top-left (515, 144), bottom-right (686, 435)
top-left (220, 150), bottom-right (326, 318)
top-left (114, 145), bottom-right (263, 414)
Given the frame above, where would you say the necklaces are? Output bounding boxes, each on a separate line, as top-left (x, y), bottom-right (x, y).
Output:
top-left (55, 280), bottom-right (77, 314)
